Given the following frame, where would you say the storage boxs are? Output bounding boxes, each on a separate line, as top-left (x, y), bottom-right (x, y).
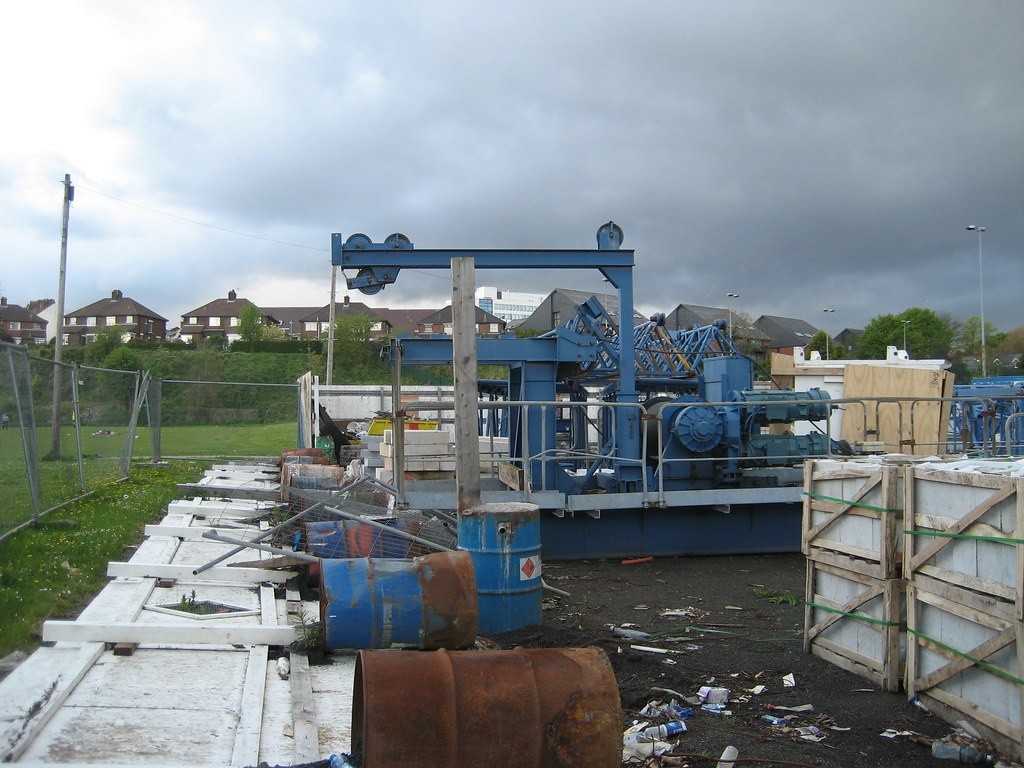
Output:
top-left (902, 456), bottom-right (1024, 621)
top-left (802, 548), bottom-right (907, 692)
top-left (904, 572), bottom-right (1024, 766)
top-left (801, 453), bottom-right (970, 579)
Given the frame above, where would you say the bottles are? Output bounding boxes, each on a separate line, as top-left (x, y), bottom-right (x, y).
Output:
top-left (636, 721), bottom-right (687, 741)
top-left (715, 746), bottom-right (738, 768)
top-left (929, 741), bottom-right (993, 764)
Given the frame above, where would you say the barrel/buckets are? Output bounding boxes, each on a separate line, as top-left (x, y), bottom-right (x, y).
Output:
top-left (279, 446), bottom-right (625, 767)
